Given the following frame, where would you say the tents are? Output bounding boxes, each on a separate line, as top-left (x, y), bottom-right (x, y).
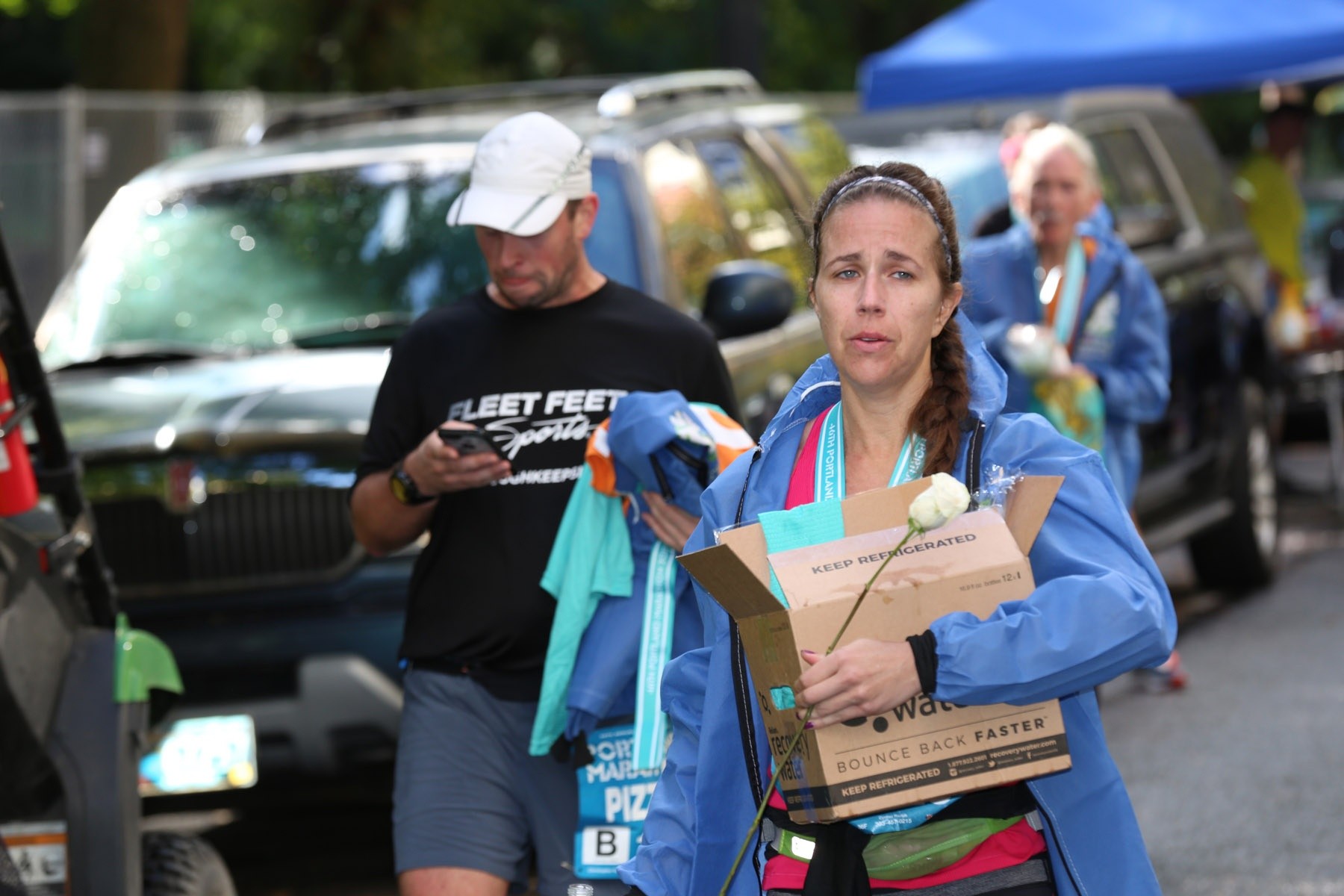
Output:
top-left (855, 0), bottom-right (1344, 116)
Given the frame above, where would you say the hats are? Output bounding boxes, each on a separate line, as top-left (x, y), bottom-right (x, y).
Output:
top-left (444, 111), bottom-right (593, 238)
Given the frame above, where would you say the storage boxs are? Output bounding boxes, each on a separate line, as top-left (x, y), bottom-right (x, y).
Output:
top-left (674, 471), bottom-right (1081, 826)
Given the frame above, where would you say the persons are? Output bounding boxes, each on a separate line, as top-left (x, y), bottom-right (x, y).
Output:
top-left (346, 110), bottom-right (744, 896)
top-left (623, 165), bottom-right (1181, 896)
top-left (959, 111), bottom-right (1172, 510)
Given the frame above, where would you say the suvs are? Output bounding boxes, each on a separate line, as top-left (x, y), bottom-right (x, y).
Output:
top-left (0, 69), bottom-right (1344, 895)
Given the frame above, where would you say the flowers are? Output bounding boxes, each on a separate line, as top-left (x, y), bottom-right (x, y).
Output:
top-left (718, 471), bottom-right (972, 896)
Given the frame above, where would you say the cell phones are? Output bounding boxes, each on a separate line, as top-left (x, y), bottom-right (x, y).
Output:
top-left (439, 429), bottom-right (516, 476)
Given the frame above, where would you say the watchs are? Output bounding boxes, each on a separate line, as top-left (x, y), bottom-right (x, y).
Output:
top-left (386, 459), bottom-right (440, 506)
